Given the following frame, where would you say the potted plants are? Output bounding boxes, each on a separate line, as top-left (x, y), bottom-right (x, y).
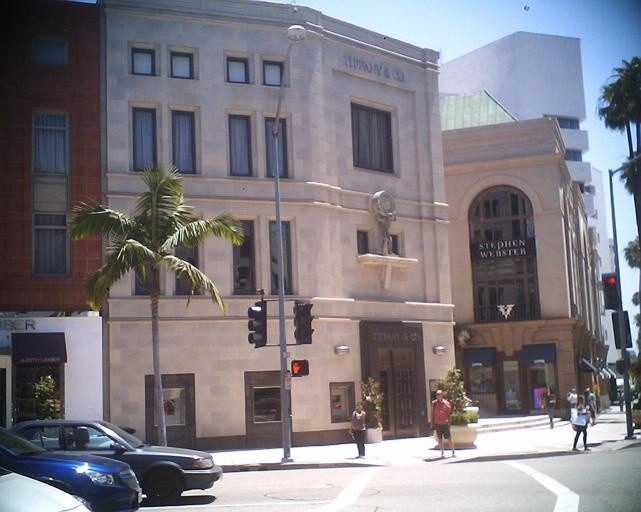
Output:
top-left (360, 377), bottom-right (384, 445)
top-left (434, 367), bottom-right (479, 448)
top-left (629, 352), bottom-right (641, 427)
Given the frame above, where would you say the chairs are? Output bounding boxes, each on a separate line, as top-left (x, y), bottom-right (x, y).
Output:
top-left (64, 432), bottom-right (75, 447)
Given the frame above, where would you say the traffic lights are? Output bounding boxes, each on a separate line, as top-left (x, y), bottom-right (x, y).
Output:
top-left (605, 274), bottom-right (621, 309)
top-left (298, 305), bottom-right (315, 344)
top-left (248, 305), bottom-right (265, 346)
top-left (291, 304), bottom-right (297, 339)
top-left (290, 359), bottom-right (310, 375)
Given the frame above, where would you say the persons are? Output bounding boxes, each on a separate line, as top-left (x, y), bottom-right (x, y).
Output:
top-left (541, 387), bottom-right (556, 430)
top-left (349, 402), bottom-right (366, 459)
top-left (567, 388), bottom-right (596, 451)
top-left (618, 392), bottom-right (625, 412)
top-left (431, 390), bottom-right (456, 458)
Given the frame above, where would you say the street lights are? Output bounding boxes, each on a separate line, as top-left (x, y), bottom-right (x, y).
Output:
top-left (273, 23), bottom-right (306, 468)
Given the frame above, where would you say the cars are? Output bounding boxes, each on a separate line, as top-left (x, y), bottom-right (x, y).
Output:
top-left (16, 396), bottom-right (52, 419)
top-left (11, 420), bottom-right (223, 501)
top-left (1, 428), bottom-right (146, 510)
top-left (1, 468), bottom-right (94, 512)
top-left (612, 376), bottom-right (637, 404)
top-left (254, 394), bottom-right (279, 420)
